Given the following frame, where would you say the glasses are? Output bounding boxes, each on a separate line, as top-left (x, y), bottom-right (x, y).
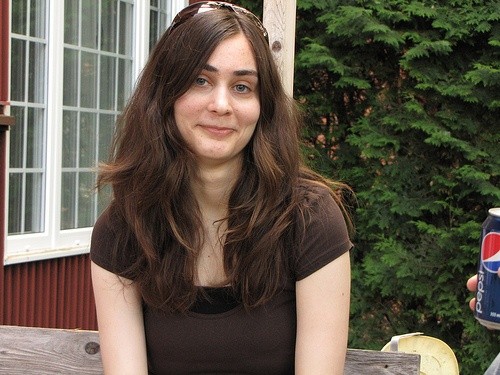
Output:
top-left (168, 1), bottom-right (269, 47)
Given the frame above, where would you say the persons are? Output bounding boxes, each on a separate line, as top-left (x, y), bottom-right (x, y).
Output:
top-left (91, 1), bottom-right (357, 375)
top-left (467, 266), bottom-right (500, 375)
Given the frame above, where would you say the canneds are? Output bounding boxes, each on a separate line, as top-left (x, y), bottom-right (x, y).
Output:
top-left (473, 207), bottom-right (500, 330)
